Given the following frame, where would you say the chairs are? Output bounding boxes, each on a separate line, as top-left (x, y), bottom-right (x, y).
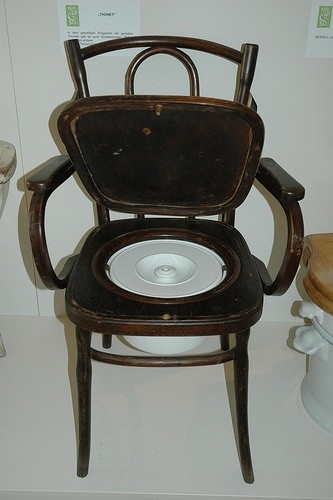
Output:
top-left (25, 34), bottom-right (307, 484)
